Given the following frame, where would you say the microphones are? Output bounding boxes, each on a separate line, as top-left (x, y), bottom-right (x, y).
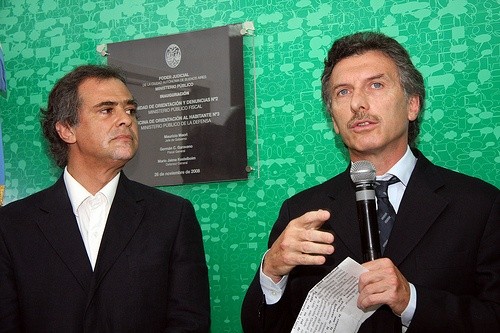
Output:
top-left (350, 160), bottom-right (382, 266)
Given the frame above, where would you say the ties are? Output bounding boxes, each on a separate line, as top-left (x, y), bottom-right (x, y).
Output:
top-left (374, 176), bottom-right (400, 258)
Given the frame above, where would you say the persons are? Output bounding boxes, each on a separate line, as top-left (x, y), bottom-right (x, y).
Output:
top-left (239, 32), bottom-right (499, 333)
top-left (0, 63), bottom-right (213, 331)
